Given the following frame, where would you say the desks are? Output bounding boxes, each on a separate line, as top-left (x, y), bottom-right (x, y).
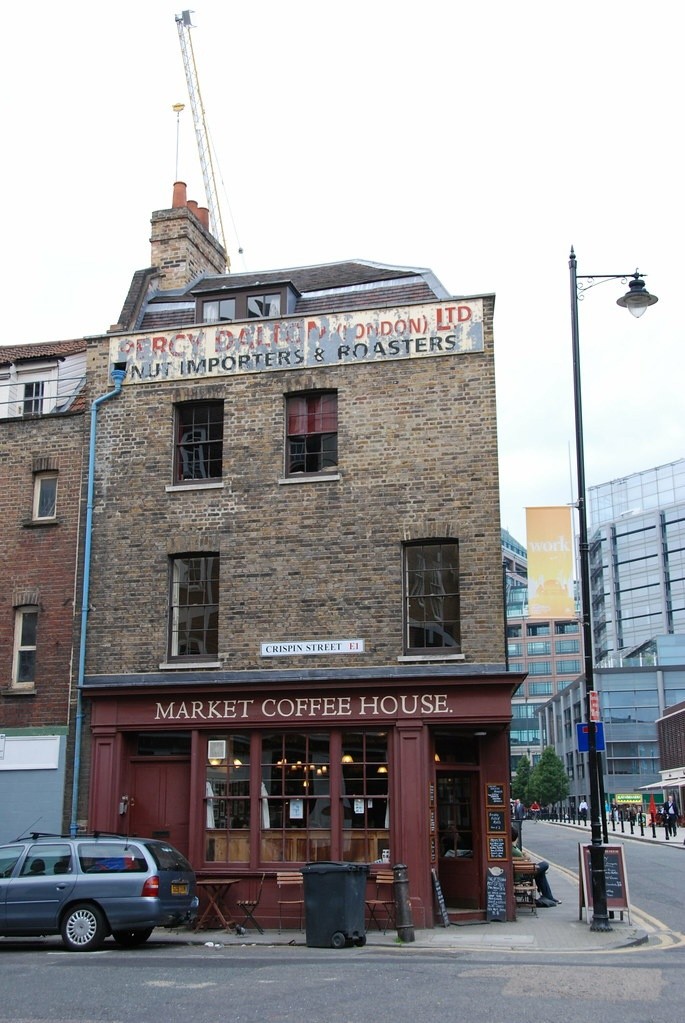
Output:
top-left (512, 861), bottom-right (536, 904)
top-left (194, 878), bottom-right (241, 934)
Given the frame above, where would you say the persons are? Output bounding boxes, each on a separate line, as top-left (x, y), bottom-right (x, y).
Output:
top-left (605, 801), bottom-right (637, 826)
top-left (579, 799), bottom-right (588, 821)
top-left (663, 796), bottom-right (679, 837)
top-left (530, 801), bottom-right (539, 819)
top-left (514, 799), bottom-right (525, 830)
top-left (511, 825), bottom-right (561, 907)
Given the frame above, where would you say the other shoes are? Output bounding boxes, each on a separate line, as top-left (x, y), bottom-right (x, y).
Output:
top-left (551, 898), bottom-right (561, 904)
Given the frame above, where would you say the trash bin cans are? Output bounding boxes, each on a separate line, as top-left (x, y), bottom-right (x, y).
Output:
top-left (300, 860), bottom-right (371, 947)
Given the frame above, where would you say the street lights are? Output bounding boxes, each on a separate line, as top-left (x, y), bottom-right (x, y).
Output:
top-left (569, 271), bottom-right (661, 935)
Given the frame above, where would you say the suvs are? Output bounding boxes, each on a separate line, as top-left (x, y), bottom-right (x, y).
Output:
top-left (0, 830), bottom-right (201, 950)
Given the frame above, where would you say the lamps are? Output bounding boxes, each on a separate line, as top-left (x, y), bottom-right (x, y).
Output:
top-left (233, 757), bottom-right (242, 768)
top-left (209, 758), bottom-right (224, 768)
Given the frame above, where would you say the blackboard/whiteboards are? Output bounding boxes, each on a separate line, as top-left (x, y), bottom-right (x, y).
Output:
top-left (486, 810), bottom-right (506, 834)
top-left (428, 839), bottom-right (438, 866)
top-left (577, 842), bottom-right (629, 912)
top-left (429, 810), bottom-right (438, 835)
top-left (486, 868), bottom-right (507, 924)
top-left (431, 868), bottom-right (450, 928)
top-left (486, 836), bottom-right (508, 861)
top-left (426, 782), bottom-right (436, 810)
top-left (484, 783), bottom-right (506, 807)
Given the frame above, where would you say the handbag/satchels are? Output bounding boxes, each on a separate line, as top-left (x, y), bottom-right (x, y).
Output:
top-left (535, 895), bottom-right (555, 908)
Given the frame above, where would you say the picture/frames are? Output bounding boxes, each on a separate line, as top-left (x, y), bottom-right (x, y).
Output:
top-left (208, 740), bottom-right (225, 759)
top-left (485, 809), bottom-right (507, 835)
top-left (485, 783), bottom-right (507, 808)
top-left (427, 779), bottom-right (437, 809)
top-left (488, 835), bottom-right (509, 863)
top-left (430, 836), bottom-right (438, 864)
top-left (428, 809), bottom-right (437, 836)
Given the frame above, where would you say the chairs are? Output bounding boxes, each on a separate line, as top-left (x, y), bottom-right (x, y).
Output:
top-left (512, 861), bottom-right (538, 918)
top-left (53, 862), bottom-right (65, 874)
top-left (365, 871), bottom-right (397, 935)
top-left (27, 858), bottom-right (46, 875)
top-left (238, 873), bottom-right (266, 936)
top-left (276, 871), bottom-right (305, 936)
